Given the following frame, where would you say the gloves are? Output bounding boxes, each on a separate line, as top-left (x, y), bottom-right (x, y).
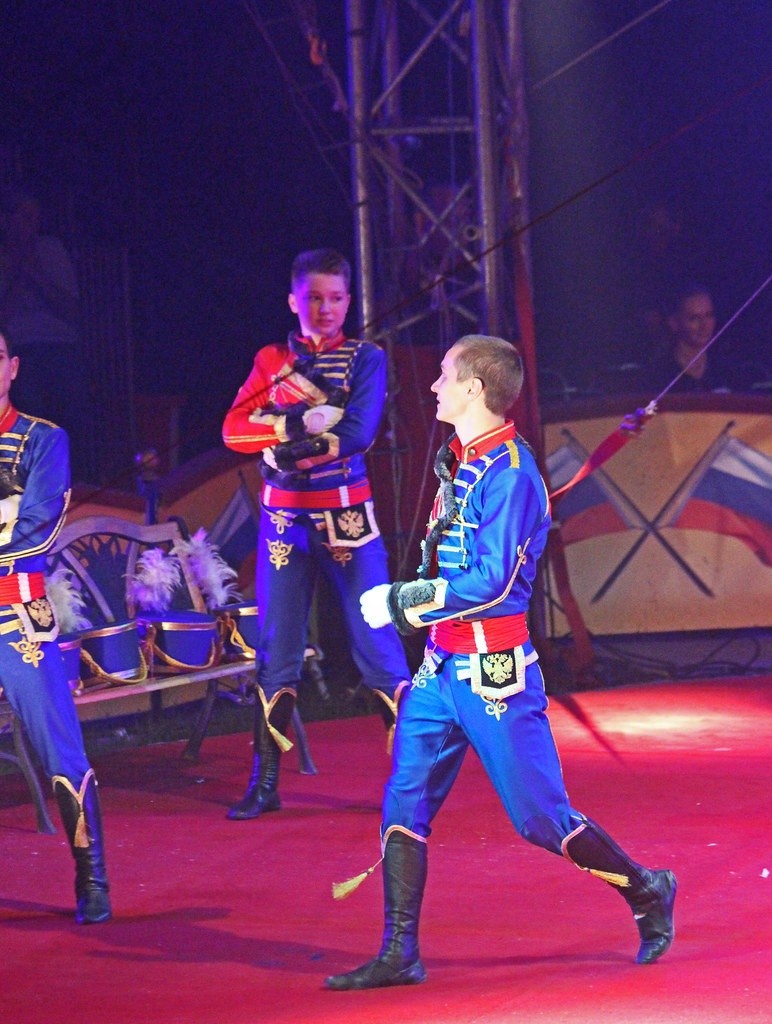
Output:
top-left (262, 445), bottom-right (282, 473)
top-left (0, 494), bottom-right (23, 525)
top-left (359, 584), bottom-right (393, 629)
top-left (302, 405), bottom-right (344, 435)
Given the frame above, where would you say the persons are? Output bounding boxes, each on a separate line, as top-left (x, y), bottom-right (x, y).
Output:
top-left (323, 336), bottom-right (677, 992)
top-left (645, 278), bottom-right (728, 394)
top-left (222, 249), bottom-right (410, 821)
top-left (0, 334), bottom-right (112, 924)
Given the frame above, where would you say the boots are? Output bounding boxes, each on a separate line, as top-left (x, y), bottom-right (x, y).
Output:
top-left (51, 768), bottom-right (112, 924)
top-left (372, 681), bottom-right (411, 757)
top-left (228, 683), bottom-right (297, 819)
top-left (561, 812), bottom-right (677, 964)
top-left (324, 825), bottom-right (427, 989)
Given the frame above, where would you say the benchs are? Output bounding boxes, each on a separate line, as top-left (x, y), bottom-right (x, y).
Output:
top-left (0, 515), bottom-right (327, 834)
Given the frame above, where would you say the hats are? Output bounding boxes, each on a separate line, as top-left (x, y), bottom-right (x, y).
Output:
top-left (44, 527), bottom-right (259, 695)
